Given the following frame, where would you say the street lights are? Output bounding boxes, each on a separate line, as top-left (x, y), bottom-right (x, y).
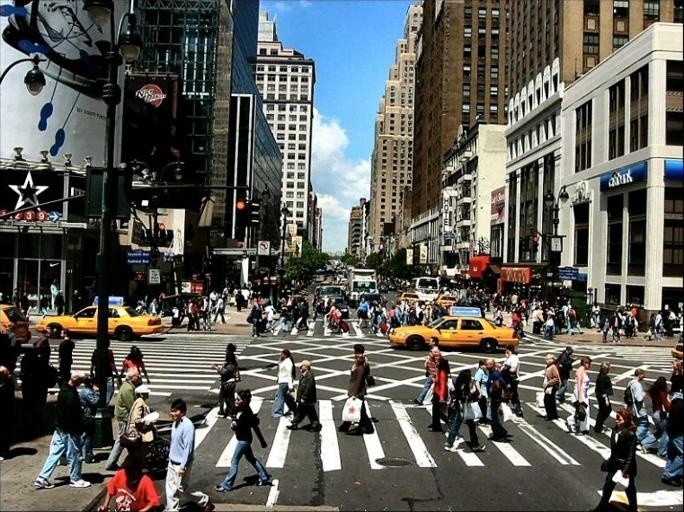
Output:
top-left (258, 183), bottom-right (270, 324)
top-left (0, 55), bottom-right (46, 97)
top-left (423, 233), bottom-right (432, 265)
top-left (278, 202), bottom-right (289, 309)
top-left (118, 159), bottom-right (185, 268)
top-left (544, 185), bottom-right (569, 328)
top-left (80, 1), bottom-right (145, 448)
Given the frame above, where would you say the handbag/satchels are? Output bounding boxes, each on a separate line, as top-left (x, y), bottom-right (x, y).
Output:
top-left (574, 405), bottom-right (586, 423)
top-left (365, 375), bottom-right (375, 386)
top-left (470, 377), bottom-right (482, 396)
top-left (463, 396), bottom-right (483, 421)
top-left (342, 397), bottom-right (363, 423)
top-left (612, 469), bottom-right (630, 488)
top-left (119, 429), bottom-right (142, 448)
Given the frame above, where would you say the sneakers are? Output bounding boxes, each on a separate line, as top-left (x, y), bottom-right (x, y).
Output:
top-left (636, 440), bottom-right (667, 460)
top-left (214, 486), bottom-right (229, 493)
top-left (444, 443), bottom-right (457, 452)
top-left (472, 445), bottom-right (486, 451)
top-left (257, 475), bottom-right (272, 486)
top-left (34, 476), bottom-right (55, 489)
top-left (661, 477), bottom-right (681, 486)
top-left (69, 479), bottom-right (90, 488)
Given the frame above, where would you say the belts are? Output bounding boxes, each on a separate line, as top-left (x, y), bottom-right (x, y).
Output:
top-left (171, 460), bottom-right (181, 465)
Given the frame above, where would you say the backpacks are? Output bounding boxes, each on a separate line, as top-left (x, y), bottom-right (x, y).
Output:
top-left (624, 387), bottom-right (633, 406)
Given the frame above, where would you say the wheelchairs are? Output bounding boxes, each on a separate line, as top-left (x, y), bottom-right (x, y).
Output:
top-left (141, 422), bottom-right (170, 480)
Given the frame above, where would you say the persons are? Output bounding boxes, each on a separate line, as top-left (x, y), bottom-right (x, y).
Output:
top-left (541, 346), bottom-right (683, 511)
top-left (213, 342), bottom-right (242, 420)
top-left (355, 298), bottom-right (450, 336)
top-left (271, 349), bottom-right (298, 418)
top-left (1, 320), bottom-right (153, 490)
top-left (135, 284), bottom-right (229, 331)
top-left (286, 360), bottom-right (323, 433)
top-left (1, 278), bottom-right (85, 317)
top-left (336, 345), bottom-right (376, 436)
top-left (165, 398), bottom-right (215, 512)
top-left (413, 337), bottom-right (523, 453)
top-left (97, 452), bottom-right (161, 512)
top-left (228, 280), bottom-right (342, 337)
top-left (450, 282), bottom-right (684, 344)
top-left (213, 389), bottom-right (272, 492)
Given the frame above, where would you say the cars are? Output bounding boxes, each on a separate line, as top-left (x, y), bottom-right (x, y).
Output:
top-left (33, 296), bottom-right (164, 341)
top-left (314, 258), bottom-right (458, 319)
top-left (388, 306), bottom-right (519, 354)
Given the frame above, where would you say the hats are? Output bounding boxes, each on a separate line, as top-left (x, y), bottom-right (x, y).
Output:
top-left (135, 384), bottom-right (151, 393)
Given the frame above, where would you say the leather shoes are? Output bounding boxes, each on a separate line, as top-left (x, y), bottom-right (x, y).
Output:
top-left (202, 498), bottom-right (211, 511)
top-left (287, 424), bottom-right (297, 430)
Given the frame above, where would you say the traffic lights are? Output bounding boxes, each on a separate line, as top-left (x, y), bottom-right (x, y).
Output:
top-left (247, 200), bottom-right (259, 228)
top-left (522, 235), bottom-right (530, 252)
top-left (236, 199), bottom-right (247, 229)
top-left (533, 235), bottom-right (539, 254)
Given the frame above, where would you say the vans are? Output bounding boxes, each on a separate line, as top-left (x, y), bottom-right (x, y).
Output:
top-left (0, 303), bottom-right (32, 345)
